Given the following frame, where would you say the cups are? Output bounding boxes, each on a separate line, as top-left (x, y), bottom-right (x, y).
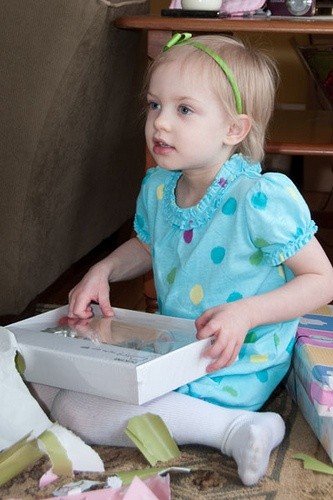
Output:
top-left (182, 0), bottom-right (222, 11)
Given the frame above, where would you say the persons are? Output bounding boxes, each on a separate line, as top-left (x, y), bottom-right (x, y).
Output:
top-left (27, 33), bottom-right (333, 486)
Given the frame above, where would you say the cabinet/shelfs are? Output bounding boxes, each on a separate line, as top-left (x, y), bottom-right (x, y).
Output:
top-left (120, 13), bottom-right (333, 300)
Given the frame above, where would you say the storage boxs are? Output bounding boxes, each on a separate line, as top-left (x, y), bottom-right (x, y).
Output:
top-left (3, 300), bottom-right (240, 406)
top-left (271, 305), bottom-right (333, 463)
